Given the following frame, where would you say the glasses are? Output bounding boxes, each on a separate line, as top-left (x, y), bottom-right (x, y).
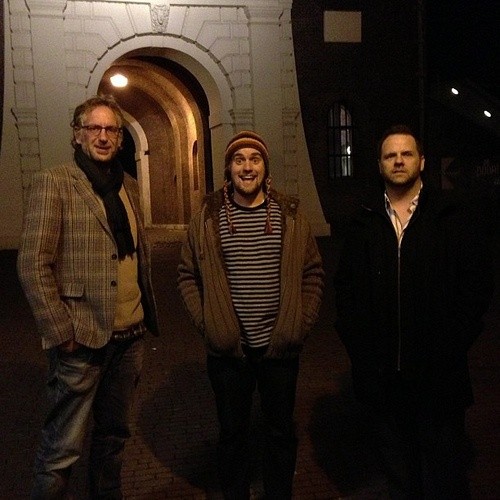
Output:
top-left (79, 123), bottom-right (123, 135)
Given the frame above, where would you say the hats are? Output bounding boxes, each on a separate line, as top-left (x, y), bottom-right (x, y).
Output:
top-left (224, 131), bottom-right (272, 234)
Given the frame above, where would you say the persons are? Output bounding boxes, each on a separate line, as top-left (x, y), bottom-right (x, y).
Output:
top-left (177, 131), bottom-right (325, 500)
top-left (342, 125), bottom-right (492, 500)
top-left (17, 98), bottom-right (159, 500)
